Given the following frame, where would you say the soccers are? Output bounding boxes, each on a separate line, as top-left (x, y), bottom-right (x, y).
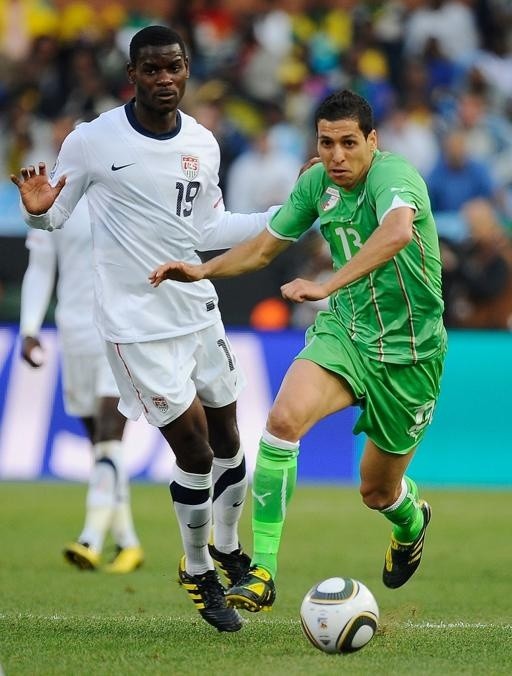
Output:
top-left (301, 578), bottom-right (379, 653)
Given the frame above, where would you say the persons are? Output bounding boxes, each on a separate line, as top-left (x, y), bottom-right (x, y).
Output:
top-left (151, 91), bottom-right (447, 612)
top-left (16, 117), bottom-right (147, 572)
top-left (10, 26), bottom-right (324, 628)
top-left (1, 0), bottom-right (512, 331)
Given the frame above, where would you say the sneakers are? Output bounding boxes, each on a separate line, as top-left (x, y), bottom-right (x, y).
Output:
top-left (106, 544), bottom-right (143, 574)
top-left (179, 526), bottom-right (277, 632)
top-left (64, 543), bottom-right (99, 569)
top-left (382, 499), bottom-right (431, 590)
